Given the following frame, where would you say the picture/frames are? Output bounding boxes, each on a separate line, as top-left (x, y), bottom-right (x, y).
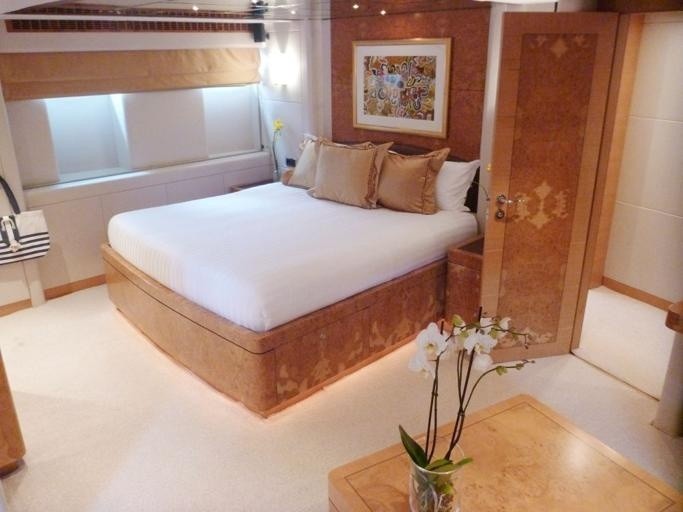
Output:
top-left (352, 37), bottom-right (452, 138)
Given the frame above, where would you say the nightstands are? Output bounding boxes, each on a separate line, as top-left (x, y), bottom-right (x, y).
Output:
top-left (444, 236), bottom-right (482, 334)
top-left (230, 177), bottom-right (273, 191)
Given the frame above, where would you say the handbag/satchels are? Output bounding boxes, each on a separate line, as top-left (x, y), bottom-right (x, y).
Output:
top-left (0, 209), bottom-right (51, 265)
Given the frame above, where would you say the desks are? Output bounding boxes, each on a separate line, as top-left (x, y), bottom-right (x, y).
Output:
top-left (328, 394), bottom-right (683, 512)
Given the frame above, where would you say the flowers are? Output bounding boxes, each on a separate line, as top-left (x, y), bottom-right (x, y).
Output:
top-left (398, 306), bottom-right (551, 473)
top-left (271, 120), bottom-right (285, 181)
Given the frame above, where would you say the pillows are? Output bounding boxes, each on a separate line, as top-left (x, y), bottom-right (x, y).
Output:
top-left (283, 134), bottom-right (323, 188)
top-left (377, 147), bottom-right (451, 214)
top-left (435, 159), bottom-right (480, 211)
top-left (307, 140), bottom-right (393, 209)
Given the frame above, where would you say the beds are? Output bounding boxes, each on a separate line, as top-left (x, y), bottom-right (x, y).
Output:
top-left (99, 140), bottom-right (479, 418)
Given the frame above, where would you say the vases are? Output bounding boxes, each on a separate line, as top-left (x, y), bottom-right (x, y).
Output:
top-left (407, 435), bottom-right (465, 512)
